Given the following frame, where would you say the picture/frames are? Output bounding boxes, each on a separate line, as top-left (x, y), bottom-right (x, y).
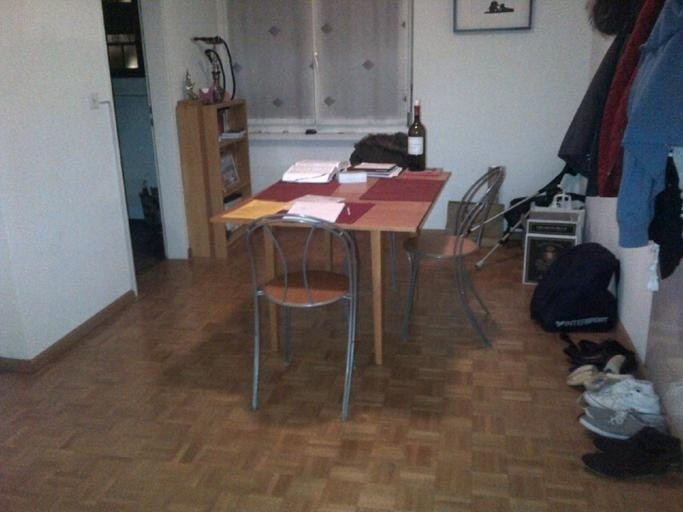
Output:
top-left (522, 218), bottom-right (581, 285)
top-left (454, 0), bottom-right (532, 32)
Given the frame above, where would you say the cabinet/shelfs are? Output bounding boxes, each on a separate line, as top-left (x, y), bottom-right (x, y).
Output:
top-left (176, 94), bottom-right (252, 258)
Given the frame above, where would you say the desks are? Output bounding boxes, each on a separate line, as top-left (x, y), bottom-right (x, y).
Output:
top-left (209, 172), bottom-right (451, 371)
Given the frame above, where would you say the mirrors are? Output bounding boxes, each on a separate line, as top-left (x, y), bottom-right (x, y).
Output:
top-left (645, 258), bottom-right (683, 452)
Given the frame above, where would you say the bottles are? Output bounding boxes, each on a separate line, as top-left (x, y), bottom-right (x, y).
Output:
top-left (406, 105), bottom-right (427, 171)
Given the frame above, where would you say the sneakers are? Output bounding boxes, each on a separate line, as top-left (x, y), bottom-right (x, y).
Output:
top-left (565, 338), bottom-right (683, 479)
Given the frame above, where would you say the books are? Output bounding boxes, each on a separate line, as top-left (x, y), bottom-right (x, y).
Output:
top-left (281, 158), bottom-right (348, 183)
top-left (351, 160), bottom-right (403, 181)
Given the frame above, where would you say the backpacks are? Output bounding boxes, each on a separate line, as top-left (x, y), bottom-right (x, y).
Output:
top-left (530, 241), bottom-right (621, 332)
